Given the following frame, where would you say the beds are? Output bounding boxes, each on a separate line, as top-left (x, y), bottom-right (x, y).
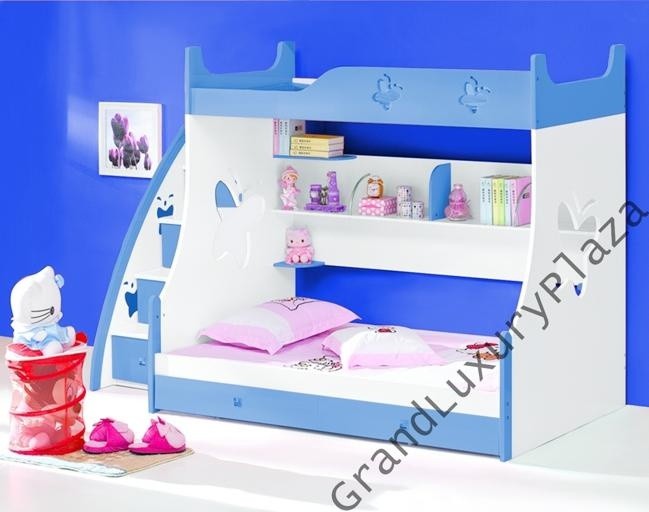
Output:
top-left (91, 41), bottom-right (628, 462)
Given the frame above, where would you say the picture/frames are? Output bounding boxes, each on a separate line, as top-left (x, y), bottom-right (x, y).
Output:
top-left (98, 102), bottom-right (162, 179)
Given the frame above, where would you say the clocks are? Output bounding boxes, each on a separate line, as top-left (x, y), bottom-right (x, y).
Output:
top-left (367, 176), bottom-right (384, 199)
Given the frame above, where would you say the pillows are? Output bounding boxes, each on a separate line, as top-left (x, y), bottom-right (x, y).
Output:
top-left (322, 324), bottom-right (445, 369)
top-left (197, 297), bottom-right (361, 355)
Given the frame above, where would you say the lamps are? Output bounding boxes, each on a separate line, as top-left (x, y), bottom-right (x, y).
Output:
top-left (348, 165), bottom-right (378, 215)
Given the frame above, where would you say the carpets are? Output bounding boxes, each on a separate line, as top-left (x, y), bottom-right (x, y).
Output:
top-left (0, 432), bottom-right (194, 477)
